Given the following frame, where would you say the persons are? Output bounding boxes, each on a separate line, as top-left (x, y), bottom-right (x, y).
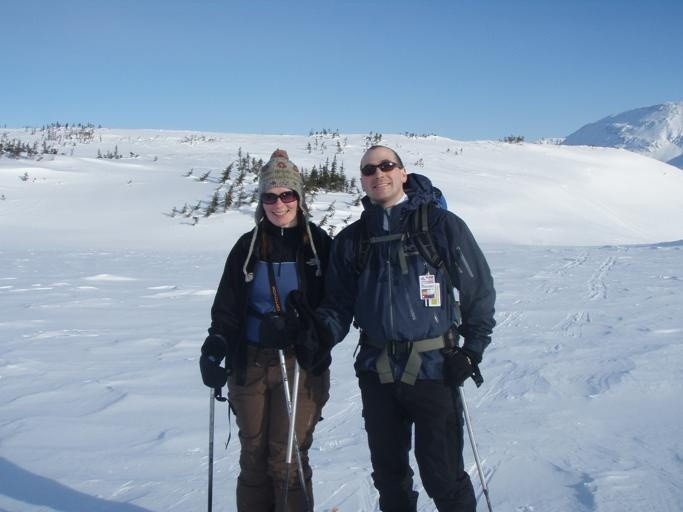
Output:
top-left (296, 146), bottom-right (495, 512)
top-left (199, 148), bottom-right (335, 511)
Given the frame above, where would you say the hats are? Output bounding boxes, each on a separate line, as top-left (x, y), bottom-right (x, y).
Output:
top-left (255, 149), bottom-right (309, 226)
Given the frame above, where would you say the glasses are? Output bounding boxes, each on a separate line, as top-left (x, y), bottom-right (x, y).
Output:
top-left (260, 191), bottom-right (299, 205)
top-left (362, 161), bottom-right (403, 176)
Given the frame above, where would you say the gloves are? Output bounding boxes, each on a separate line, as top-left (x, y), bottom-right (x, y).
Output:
top-left (444, 349), bottom-right (484, 388)
top-left (258, 311), bottom-right (294, 349)
top-left (199, 327), bottom-right (230, 389)
